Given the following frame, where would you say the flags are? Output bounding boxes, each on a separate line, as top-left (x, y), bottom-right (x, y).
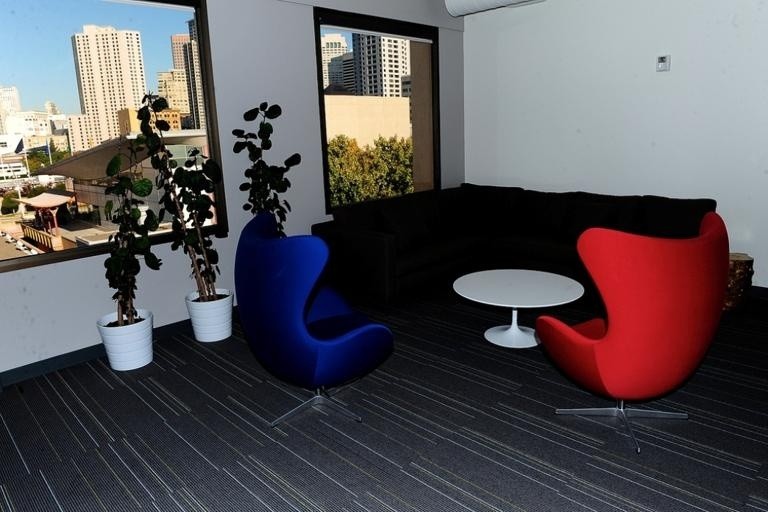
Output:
top-left (14, 138), bottom-right (25, 155)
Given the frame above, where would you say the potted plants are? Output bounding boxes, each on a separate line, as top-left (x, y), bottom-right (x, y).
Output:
top-left (96, 134), bottom-right (160, 371)
top-left (137, 90), bottom-right (234, 342)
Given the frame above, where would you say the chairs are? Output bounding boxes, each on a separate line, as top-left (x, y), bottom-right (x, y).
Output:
top-left (235, 211), bottom-right (394, 428)
top-left (535, 210), bottom-right (730, 454)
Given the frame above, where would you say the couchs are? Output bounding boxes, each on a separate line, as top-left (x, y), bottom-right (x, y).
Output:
top-left (311, 183), bottom-right (716, 324)
